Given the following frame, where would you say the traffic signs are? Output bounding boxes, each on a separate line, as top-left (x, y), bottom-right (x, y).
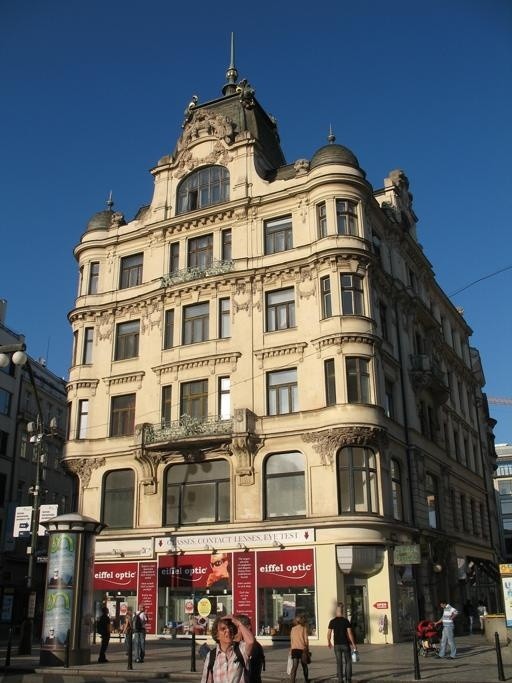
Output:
top-left (373, 602), bottom-right (388, 609)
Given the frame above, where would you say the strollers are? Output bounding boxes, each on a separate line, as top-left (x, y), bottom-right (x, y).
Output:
top-left (416, 620), bottom-right (443, 658)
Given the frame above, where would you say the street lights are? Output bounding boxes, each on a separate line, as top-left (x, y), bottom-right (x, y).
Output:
top-left (0, 350), bottom-right (59, 655)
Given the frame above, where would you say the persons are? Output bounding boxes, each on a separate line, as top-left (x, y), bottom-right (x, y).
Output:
top-left (235, 615), bottom-right (265, 682)
top-left (206, 552), bottom-right (231, 591)
top-left (289, 613), bottom-right (312, 683)
top-left (463, 598), bottom-right (476, 635)
top-left (50, 567), bottom-right (59, 584)
top-left (132, 603), bottom-right (148, 662)
top-left (201, 614), bottom-right (256, 682)
top-left (476, 598), bottom-right (487, 633)
top-left (46, 628), bottom-right (55, 644)
top-left (432, 600), bottom-right (458, 659)
top-left (121, 609), bottom-right (133, 655)
top-left (97, 606), bottom-right (112, 662)
top-left (326, 605), bottom-right (358, 683)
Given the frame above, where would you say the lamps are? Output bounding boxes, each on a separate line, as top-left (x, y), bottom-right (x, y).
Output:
top-left (202, 544), bottom-right (216, 554)
top-left (168, 544), bottom-right (183, 554)
top-left (236, 543), bottom-right (249, 552)
top-left (112, 549), bottom-right (124, 557)
top-left (273, 538), bottom-right (285, 549)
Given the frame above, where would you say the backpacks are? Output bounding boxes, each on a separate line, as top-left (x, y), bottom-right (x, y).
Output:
top-left (131, 611), bottom-right (144, 632)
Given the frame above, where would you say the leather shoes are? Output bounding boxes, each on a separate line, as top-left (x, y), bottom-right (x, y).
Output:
top-left (135, 658), bottom-right (144, 663)
top-left (435, 656), bottom-right (455, 660)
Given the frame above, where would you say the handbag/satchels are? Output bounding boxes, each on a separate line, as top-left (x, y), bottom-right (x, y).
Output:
top-left (300, 648), bottom-right (312, 664)
top-left (351, 649), bottom-right (360, 662)
